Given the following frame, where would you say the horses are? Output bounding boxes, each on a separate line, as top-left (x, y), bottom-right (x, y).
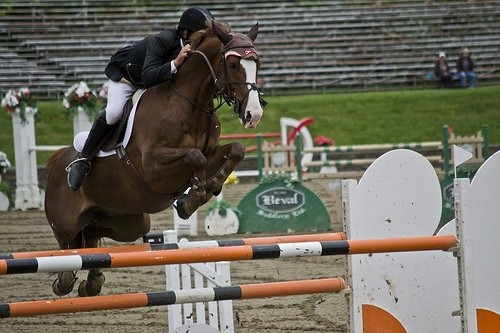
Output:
top-left (44, 17), bottom-right (267, 296)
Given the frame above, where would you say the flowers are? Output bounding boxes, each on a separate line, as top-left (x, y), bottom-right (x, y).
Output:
top-left (2, 87), bottom-right (38, 125)
top-left (224, 172), bottom-right (241, 185)
top-left (62, 81), bottom-right (99, 124)
top-left (314, 134), bottom-right (332, 146)
top-left (0, 151), bottom-right (11, 174)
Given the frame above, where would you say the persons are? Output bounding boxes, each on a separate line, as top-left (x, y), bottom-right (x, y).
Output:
top-left (458, 48), bottom-right (476, 90)
top-left (67, 5), bottom-right (214, 192)
top-left (435, 52), bottom-right (455, 88)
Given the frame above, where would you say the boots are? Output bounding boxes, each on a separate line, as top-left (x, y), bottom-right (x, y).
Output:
top-left (66, 108), bottom-right (122, 191)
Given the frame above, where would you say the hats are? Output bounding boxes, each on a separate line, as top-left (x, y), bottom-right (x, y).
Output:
top-left (439, 52), bottom-right (446, 58)
top-left (464, 48), bottom-right (468, 52)
top-left (178, 6), bottom-right (215, 32)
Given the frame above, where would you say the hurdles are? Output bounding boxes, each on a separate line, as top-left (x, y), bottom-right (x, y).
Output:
top-left (256, 125), bottom-right (484, 184)
top-left (0, 150), bottom-right (500, 332)
top-left (219, 115), bottom-right (311, 182)
top-left (7, 99), bottom-right (90, 209)
